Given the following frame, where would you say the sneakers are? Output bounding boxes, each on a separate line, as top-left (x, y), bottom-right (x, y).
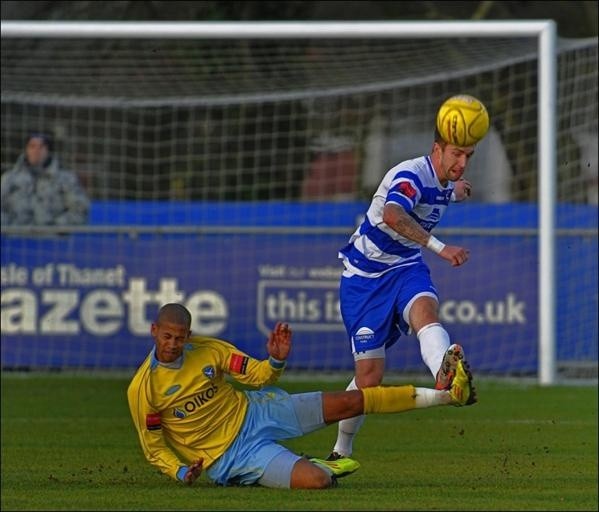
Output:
top-left (434, 343), bottom-right (477, 408)
top-left (305, 453), bottom-right (360, 489)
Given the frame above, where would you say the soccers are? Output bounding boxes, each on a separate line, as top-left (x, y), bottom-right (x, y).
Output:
top-left (436, 95), bottom-right (489, 147)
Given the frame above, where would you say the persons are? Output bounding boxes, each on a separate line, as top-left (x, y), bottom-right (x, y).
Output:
top-left (0, 127), bottom-right (91, 230)
top-left (127, 303), bottom-right (477, 491)
top-left (298, 109), bottom-right (517, 203)
top-left (327, 119), bottom-right (476, 462)
top-left (573, 128), bottom-right (598, 205)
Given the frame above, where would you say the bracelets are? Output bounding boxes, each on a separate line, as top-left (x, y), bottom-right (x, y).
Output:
top-left (427, 235), bottom-right (446, 254)
